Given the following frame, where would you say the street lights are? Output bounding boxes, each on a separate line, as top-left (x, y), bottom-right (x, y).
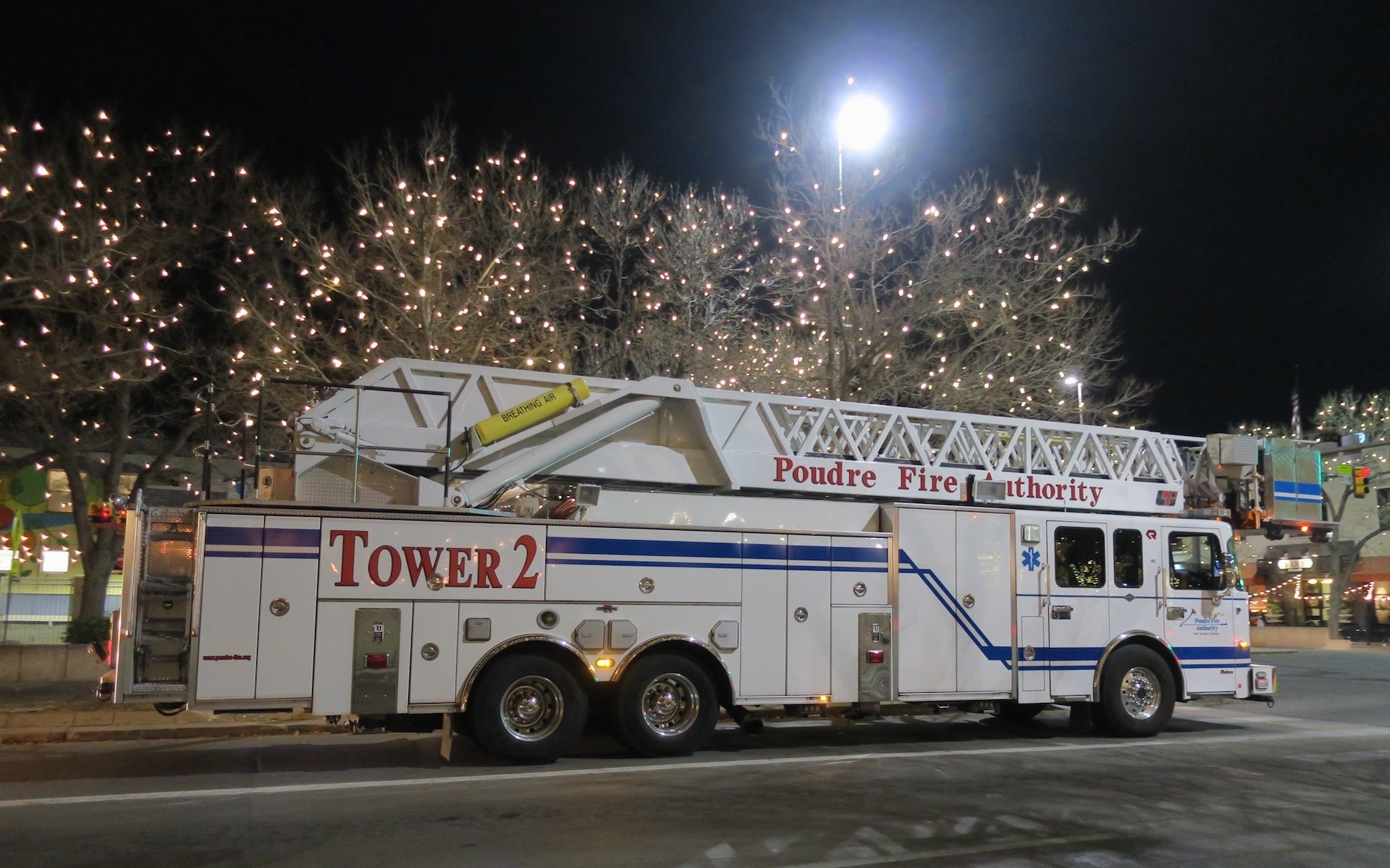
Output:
top-left (831, 77), bottom-right (892, 397)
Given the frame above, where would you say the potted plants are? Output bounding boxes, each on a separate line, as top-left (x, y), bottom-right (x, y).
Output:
top-left (1263, 602), bottom-right (1284, 623)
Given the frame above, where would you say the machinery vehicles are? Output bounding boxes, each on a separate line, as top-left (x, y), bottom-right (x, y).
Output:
top-left (86, 357), bottom-right (1341, 766)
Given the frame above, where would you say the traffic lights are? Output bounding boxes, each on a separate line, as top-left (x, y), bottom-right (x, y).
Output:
top-left (1354, 465), bottom-right (1371, 498)
